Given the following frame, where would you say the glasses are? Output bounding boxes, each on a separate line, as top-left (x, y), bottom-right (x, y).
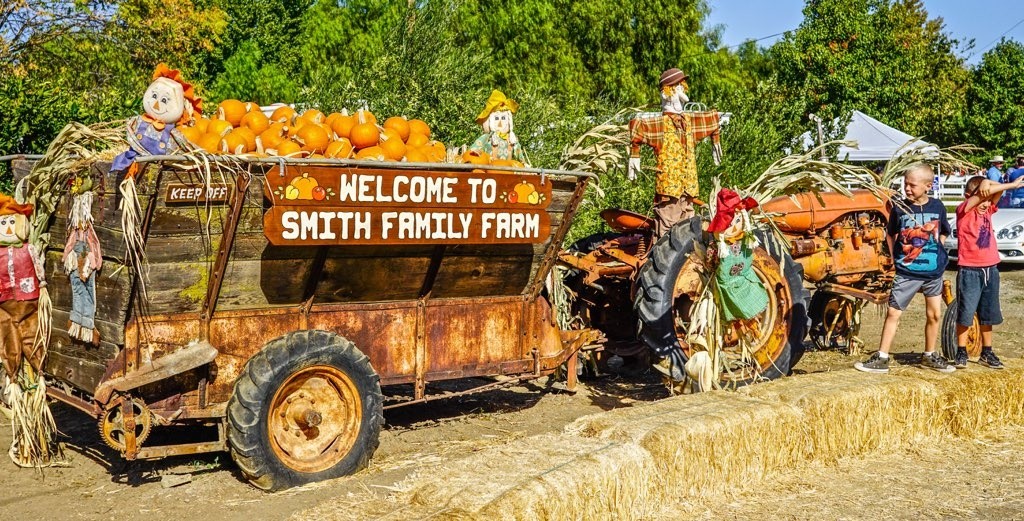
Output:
top-left (996, 161), bottom-right (1004, 164)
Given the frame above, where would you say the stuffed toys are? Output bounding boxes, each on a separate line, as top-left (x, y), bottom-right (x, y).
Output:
top-left (111, 62), bottom-right (202, 176)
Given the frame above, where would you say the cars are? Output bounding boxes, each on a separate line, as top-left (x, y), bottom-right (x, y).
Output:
top-left (945, 186), bottom-right (1024, 266)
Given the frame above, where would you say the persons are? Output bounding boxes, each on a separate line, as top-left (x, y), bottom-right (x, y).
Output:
top-left (627, 68), bottom-right (732, 242)
top-left (854, 163), bottom-right (956, 373)
top-left (470, 92), bottom-right (530, 171)
top-left (0, 194), bottom-right (52, 398)
top-left (955, 154), bottom-right (1024, 368)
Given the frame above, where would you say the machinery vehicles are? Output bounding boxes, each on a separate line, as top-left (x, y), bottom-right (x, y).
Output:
top-left (8, 146), bottom-right (893, 497)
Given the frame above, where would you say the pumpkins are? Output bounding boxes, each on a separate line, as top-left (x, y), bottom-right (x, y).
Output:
top-left (174, 99), bottom-right (526, 174)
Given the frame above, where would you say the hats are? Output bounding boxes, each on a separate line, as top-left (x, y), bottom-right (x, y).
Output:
top-left (659, 67), bottom-right (690, 87)
top-left (989, 156), bottom-right (1006, 162)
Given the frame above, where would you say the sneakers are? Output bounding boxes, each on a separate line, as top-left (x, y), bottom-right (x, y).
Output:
top-left (978, 351), bottom-right (1004, 369)
top-left (920, 352), bottom-right (957, 372)
top-left (853, 351), bottom-right (889, 373)
top-left (954, 351), bottom-right (968, 368)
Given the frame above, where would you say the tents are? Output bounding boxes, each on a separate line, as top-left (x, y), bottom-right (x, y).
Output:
top-left (785, 109), bottom-right (942, 201)
